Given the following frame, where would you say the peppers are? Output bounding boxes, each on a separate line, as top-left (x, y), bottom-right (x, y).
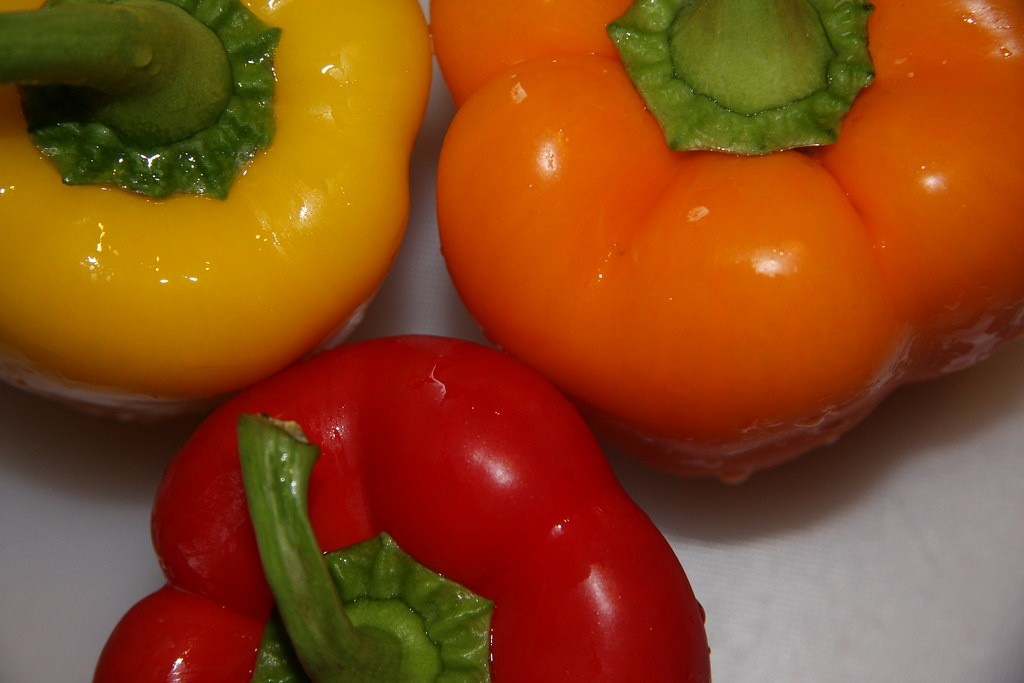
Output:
top-left (92, 333), bottom-right (709, 682)
top-left (0, 0), bottom-right (433, 424)
top-left (428, 1), bottom-right (1024, 487)
